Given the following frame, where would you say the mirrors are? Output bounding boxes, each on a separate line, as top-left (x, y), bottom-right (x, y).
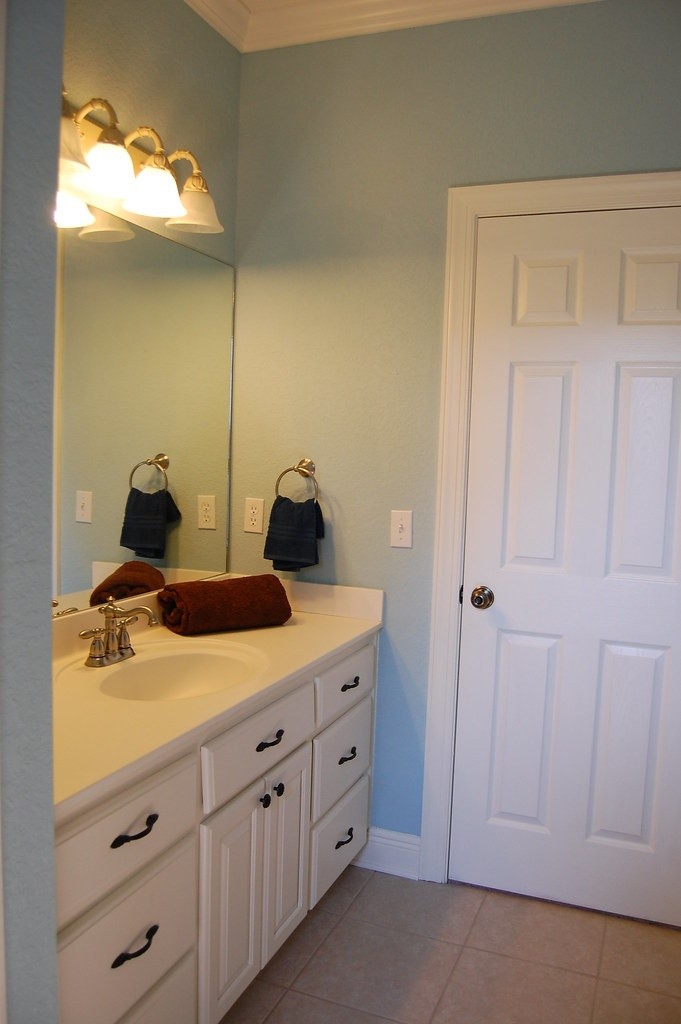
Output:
top-left (52, 204), bottom-right (235, 618)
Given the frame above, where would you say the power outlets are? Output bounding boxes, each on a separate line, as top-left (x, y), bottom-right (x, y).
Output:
top-left (245, 498), bottom-right (265, 535)
top-left (198, 495), bottom-right (216, 528)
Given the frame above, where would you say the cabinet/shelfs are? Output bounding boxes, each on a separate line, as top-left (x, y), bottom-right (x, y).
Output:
top-left (57, 633), bottom-right (378, 1024)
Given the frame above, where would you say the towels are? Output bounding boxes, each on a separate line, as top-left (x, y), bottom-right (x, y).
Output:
top-left (157, 574), bottom-right (292, 636)
top-left (88, 560), bottom-right (165, 606)
top-left (118, 487), bottom-right (182, 559)
top-left (262, 494), bottom-right (325, 573)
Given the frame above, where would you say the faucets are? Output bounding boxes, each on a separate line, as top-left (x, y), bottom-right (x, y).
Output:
top-left (52, 597), bottom-right (79, 618)
top-left (97, 605), bottom-right (160, 651)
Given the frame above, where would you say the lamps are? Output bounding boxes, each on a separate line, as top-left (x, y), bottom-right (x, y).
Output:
top-left (62, 89), bottom-right (224, 234)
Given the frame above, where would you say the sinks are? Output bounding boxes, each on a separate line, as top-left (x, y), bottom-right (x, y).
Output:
top-left (97, 653), bottom-right (249, 703)
